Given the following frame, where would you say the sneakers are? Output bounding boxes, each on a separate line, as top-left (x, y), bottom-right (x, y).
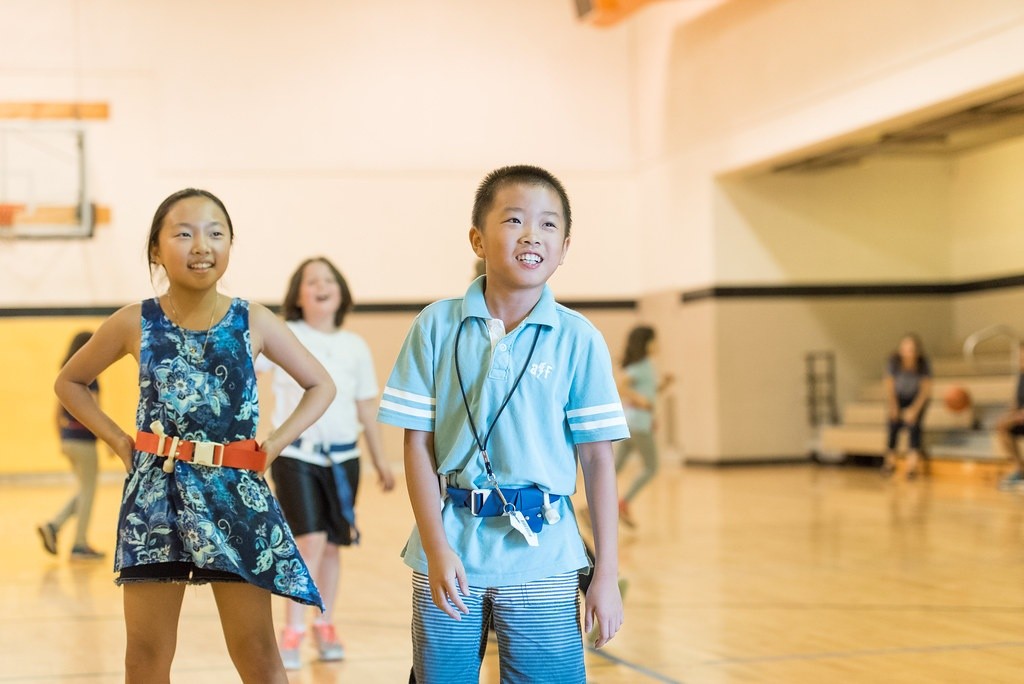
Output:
top-left (279, 626), bottom-right (306, 670)
top-left (313, 625), bottom-right (344, 660)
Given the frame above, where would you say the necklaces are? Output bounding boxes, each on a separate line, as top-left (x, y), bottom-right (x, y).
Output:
top-left (166, 285), bottom-right (222, 362)
top-left (450, 317), bottom-right (542, 548)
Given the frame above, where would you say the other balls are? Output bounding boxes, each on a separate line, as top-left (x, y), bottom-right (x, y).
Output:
top-left (943, 386), bottom-right (968, 410)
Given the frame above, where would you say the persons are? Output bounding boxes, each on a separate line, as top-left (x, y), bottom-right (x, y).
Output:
top-left (991, 341), bottom-right (1024, 479)
top-left (238, 256), bottom-right (396, 671)
top-left (880, 334), bottom-right (936, 477)
top-left (576, 322), bottom-right (674, 529)
top-left (378, 166), bottom-right (634, 684)
top-left (38, 333), bottom-right (109, 562)
top-left (53, 188), bottom-right (273, 559)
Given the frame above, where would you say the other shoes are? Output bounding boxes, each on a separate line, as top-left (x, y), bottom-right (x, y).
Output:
top-left (39, 523), bottom-right (57, 554)
top-left (72, 545), bottom-right (105, 559)
